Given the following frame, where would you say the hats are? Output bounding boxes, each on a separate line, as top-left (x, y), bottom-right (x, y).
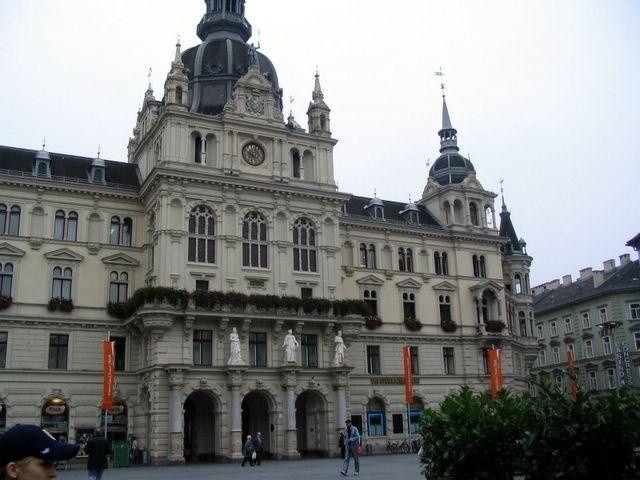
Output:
top-left (1, 422), bottom-right (81, 468)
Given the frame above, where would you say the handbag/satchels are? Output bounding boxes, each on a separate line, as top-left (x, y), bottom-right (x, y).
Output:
top-left (252, 449), bottom-right (257, 460)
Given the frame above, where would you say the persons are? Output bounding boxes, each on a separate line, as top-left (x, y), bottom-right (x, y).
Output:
top-left (0, 423), bottom-right (81, 480)
top-left (340, 419), bottom-right (361, 476)
top-left (330, 330), bottom-right (349, 367)
top-left (338, 431), bottom-right (346, 459)
top-left (253, 432), bottom-right (265, 466)
top-left (282, 329), bottom-right (299, 362)
top-left (130, 437), bottom-right (140, 466)
top-left (240, 434), bottom-right (255, 467)
top-left (84, 428), bottom-right (112, 479)
top-left (225, 326), bottom-right (244, 365)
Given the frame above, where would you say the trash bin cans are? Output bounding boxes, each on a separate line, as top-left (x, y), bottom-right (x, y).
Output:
top-left (115, 442), bottom-right (130, 467)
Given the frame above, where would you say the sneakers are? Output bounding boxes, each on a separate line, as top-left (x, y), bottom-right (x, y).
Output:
top-left (341, 470), bottom-right (348, 477)
top-left (354, 472), bottom-right (359, 476)
top-left (240, 463), bottom-right (261, 466)
